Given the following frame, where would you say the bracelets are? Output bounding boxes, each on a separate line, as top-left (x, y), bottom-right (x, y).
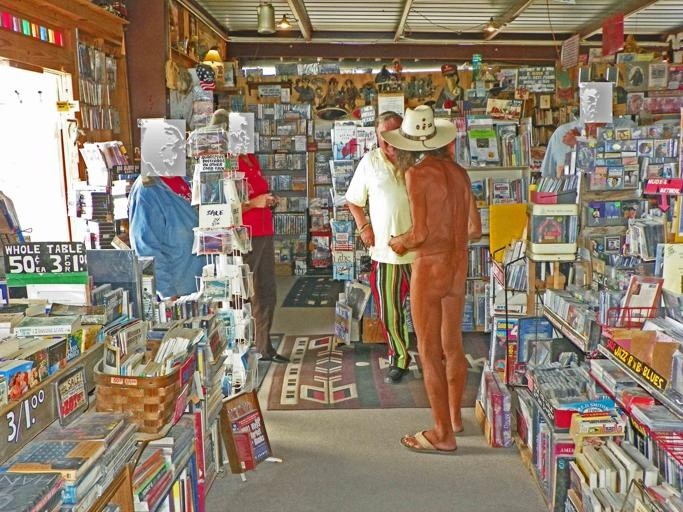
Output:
top-left (359, 222), bottom-right (369, 235)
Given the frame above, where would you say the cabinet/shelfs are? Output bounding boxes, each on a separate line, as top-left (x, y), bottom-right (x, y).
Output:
top-left (331, 119), bottom-right (520, 340)
top-left (308, 143), bottom-right (335, 276)
top-left (74, 26), bottom-right (136, 179)
top-left (527, 56), bottom-right (682, 167)
top-left (249, 102), bottom-right (313, 276)
top-left (85, 383), bottom-right (198, 511)
top-left (521, 187), bottom-right (683, 512)
top-left (193, 168), bottom-right (258, 405)
top-left (121, 0), bottom-right (231, 161)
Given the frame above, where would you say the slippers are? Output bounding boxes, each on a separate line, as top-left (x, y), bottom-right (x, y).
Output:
top-left (398, 430), bottom-right (457, 455)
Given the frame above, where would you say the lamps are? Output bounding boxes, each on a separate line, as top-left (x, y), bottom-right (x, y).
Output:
top-left (481, 17), bottom-right (503, 32)
top-left (275, 14), bottom-right (293, 29)
top-left (254, 0), bottom-right (277, 36)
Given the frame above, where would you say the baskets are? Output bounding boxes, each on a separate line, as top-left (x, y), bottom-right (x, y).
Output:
top-left (93, 339), bottom-right (194, 434)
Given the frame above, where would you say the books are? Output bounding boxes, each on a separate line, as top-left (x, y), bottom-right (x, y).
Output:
top-left (329, 122), bottom-right (389, 349)
top-left (77, 41), bottom-right (119, 132)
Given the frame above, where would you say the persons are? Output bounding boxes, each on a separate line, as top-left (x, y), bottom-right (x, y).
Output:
top-left (227, 152), bottom-right (293, 364)
top-left (540, 118), bottom-right (640, 180)
top-left (344, 111), bottom-right (419, 385)
top-left (126, 173), bottom-right (219, 302)
top-left (582, 84), bottom-right (609, 123)
top-left (389, 106), bottom-right (482, 456)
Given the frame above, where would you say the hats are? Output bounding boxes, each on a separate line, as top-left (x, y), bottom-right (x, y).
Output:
top-left (379, 104), bottom-right (456, 152)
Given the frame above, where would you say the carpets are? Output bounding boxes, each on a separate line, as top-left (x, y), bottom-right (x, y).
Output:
top-left (279, 267), bottom-right (340, 308)
top-left (266, 331), bottom-right (489, 412)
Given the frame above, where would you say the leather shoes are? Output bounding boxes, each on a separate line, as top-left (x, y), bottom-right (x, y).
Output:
top-left (259, 354), bottom-right (289, 363)
top-left (384, 367), bottom-right (408, 384)
